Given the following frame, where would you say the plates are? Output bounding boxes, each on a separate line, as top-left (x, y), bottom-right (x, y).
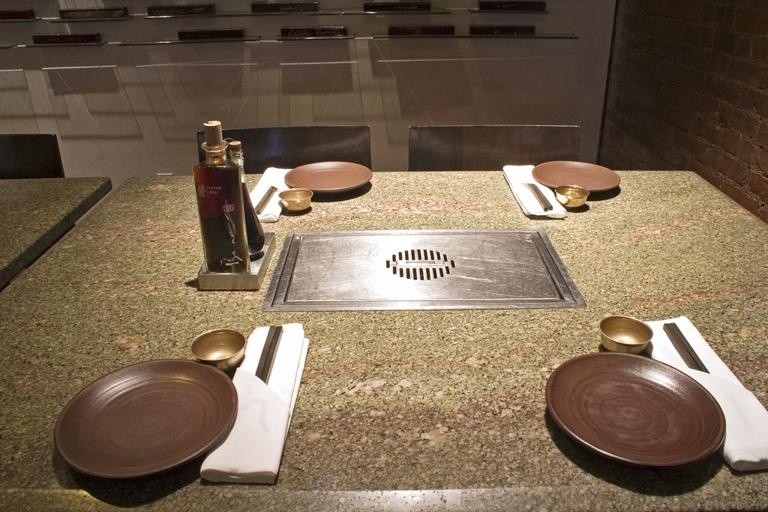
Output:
top-left (284, 161), bottom-right (373, 192)
top-left (533, 159), bottom-right (621, 193)
top-left (53, 360), bottom-right (238, 479)
top-left (546, 352), bottom-right (728, 468)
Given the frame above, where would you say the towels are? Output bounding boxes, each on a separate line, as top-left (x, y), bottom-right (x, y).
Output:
top-left (248, 165), bottom-right (294, 223)
top-left (503, 164), bottom-right (568, 220)
top-left (642, 315), bottom-right (768, 472)
top-left (201, 322), bottom-right (310, 484)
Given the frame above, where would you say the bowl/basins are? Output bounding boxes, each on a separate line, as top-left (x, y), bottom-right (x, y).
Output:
top-left (279, 189), bottom-right (313, 211)
top-left (190, 329), bottom-right (245, 368)
top-left (554, 185), bottom-right (590, 208)
top-left (600, 316), bottom-right (654, 353)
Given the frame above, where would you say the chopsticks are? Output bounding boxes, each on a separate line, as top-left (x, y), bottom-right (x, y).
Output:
top-left (528, 184), bottom-right (553, 211)
top-left (255, 186), bottom-right (277, 214)
top-left (664, 322), bottom-right (708, 372)
top-left (254, 326), bottom-right (285, 383)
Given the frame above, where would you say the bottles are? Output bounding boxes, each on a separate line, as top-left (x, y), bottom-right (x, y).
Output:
top-left (229, 141), bottom-right (265, 262)
top-left (193, 120), bottom-right (249, 273)
